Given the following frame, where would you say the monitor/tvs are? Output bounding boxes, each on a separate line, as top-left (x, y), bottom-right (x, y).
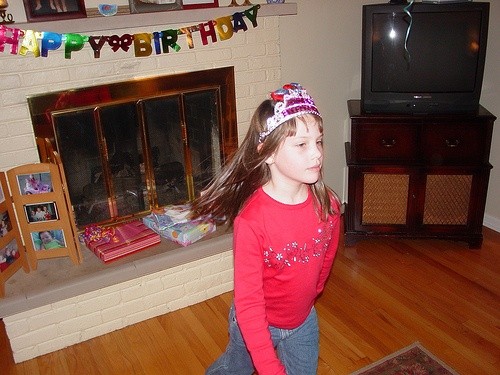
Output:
top-left (361, 2), bottom-right (490, 113)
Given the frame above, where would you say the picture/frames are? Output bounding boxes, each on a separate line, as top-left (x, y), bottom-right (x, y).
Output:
top-left (22, 0), bottom-right (88, 23)
top-left (129, 0), bottom-right (183, 13)
top-left (181, 0), bottom-right (219, 10)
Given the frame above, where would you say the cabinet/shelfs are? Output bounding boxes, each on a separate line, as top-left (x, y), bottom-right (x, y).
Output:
top-left (341, 99), bottom-right (497, 250)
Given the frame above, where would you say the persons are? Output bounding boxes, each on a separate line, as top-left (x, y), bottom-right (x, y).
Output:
top-left (0, 248), bottom-right (20, 272)
top-left (31, 206), bottom-right (51, 222)
top-left (39, 230), bottom-right (63, 251)
top-left (1, 220), bottom-right (8, 237)
top-left (29, 174), bottom-right (38, 189)
top-left (186, 83), bottom-right (341, 375)
